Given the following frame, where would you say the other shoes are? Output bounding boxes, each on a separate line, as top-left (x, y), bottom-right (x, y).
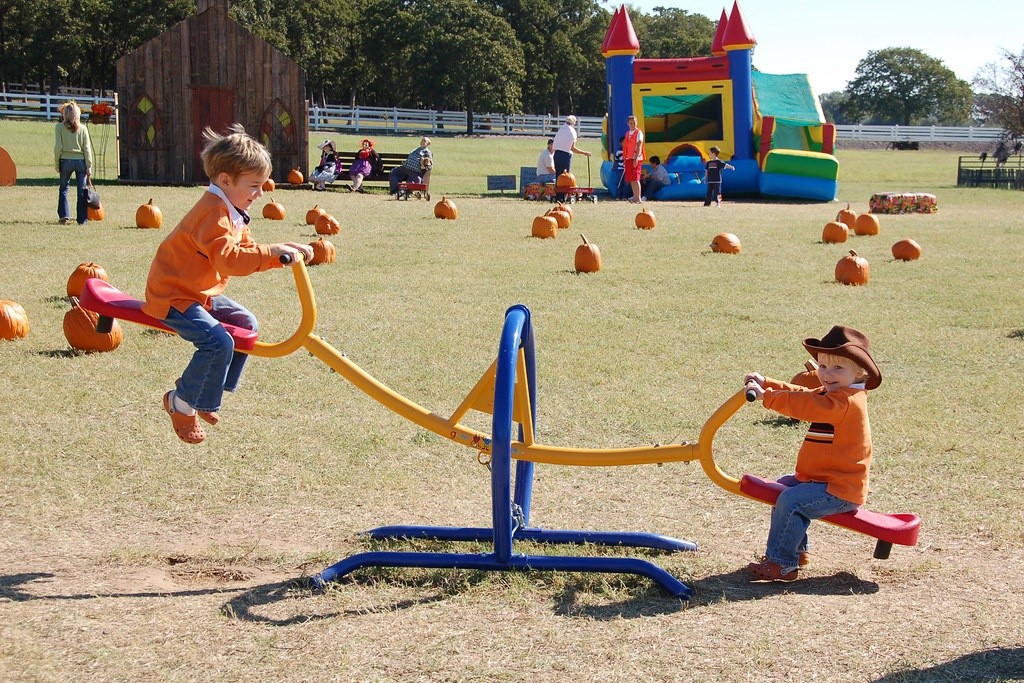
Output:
top-left (526, 193), bottom-right (535, 200)
top-left (346, 183), bottom-right (355, 192)
top-left (641, 196), bottom-right (647, 202)
top-left (545, 194), bottom-right (551, 202)
top-left (312, 186), bottom-right (325, 191)
top-left (629, 199), bottom-right (641, 204)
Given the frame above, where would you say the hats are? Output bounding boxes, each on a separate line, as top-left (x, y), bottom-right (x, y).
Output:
top-left (801, 325), bottom-right (882, 390)
top-left (318, 140), bottom-right (336, 151)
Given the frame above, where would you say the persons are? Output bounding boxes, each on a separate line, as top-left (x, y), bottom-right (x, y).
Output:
top-left (346, 140), bottom-right (379, 191)
top-left (551, 114), bottom-right (591, 202)
top-left (614, 138), bottom-right (631, 201)
top-left (528, 139), bottom-right (556, 201)
top-left (641, 156), bottom-right (671, 201)
top-left (622, 116), bottom-right (646, 203)
top-left (704, 147), bottom-right (735, 208)
top-left (743, 326), bottom-right (874, 585)
top-left (309, 140), bottom-right (342, 191)
top-left (382, 137), bottom-right (433, 200)
top-left (54, 102), bottom-right (93, 225)
top-left (145, 126), bottom-right (315, 442)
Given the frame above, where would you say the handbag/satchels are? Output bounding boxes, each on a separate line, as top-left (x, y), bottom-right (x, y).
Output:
top-left (83, 174), bottom-right (101, 210)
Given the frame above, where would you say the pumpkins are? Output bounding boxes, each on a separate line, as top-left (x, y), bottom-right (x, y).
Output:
top-left (87, 204), bottom-right (105, 220)
top-left (63, 296), bottom-right (123, 352)
top-left (835, 249), bottom-right (871, 286)
top-left (709, 233), bottom-right (740, 254)
top-left (261, 166), bottom-right (340, 266)
top-left (434, 196), bottom-right (458, 220)
top-left (136, 198), bottom-right (162, 229)
top-left (557, 169), bottom-right (575, 187)
top-left (574, 233), bottom-right (601, 272)
top-left (532, 201), bottom-right (573, 238)
top-left (822, 203), bottom-right (880, 243)
top-left (892, 238), bottom-right (921, 261)
top-left (0, 299), bottom-right (30, 341)
top-left (634, 208), bottom-right (656, 229)
top-left (66, 262), bottom-right (109, 300)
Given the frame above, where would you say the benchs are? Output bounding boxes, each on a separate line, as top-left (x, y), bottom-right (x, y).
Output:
top-left (313, 150), bottom-right (433, 194)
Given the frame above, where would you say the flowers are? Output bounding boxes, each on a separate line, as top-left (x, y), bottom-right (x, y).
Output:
top-left (91, 103), bottom-right (113, 115)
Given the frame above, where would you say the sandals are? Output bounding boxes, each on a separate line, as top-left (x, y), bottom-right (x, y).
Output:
top-left (164, 390), bottom-right (206, 444)
top-left (174, 375), bottom-right (219, 425)
top-left (747, 561), bottom-right (798, 580)
top-left (797, 551), bottom-right (809, 566)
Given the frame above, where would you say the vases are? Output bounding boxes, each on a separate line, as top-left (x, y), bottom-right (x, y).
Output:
top-left (91, 114), bottom-right (108, 124)
